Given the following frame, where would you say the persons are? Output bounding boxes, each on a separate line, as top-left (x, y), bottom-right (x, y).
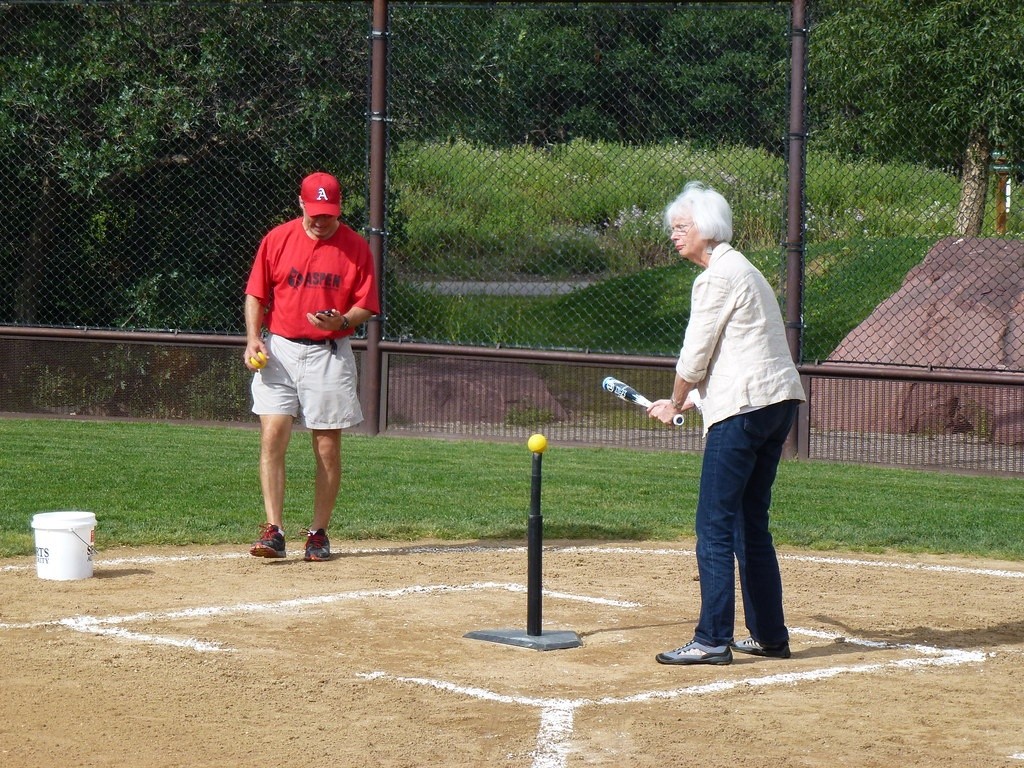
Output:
top-left (644, 181), bottom-right (807, 663)
top-left (242, 173), bottom-right (380, 562)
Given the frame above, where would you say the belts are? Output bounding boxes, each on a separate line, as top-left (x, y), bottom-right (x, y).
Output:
top-left (288, 337), bottom-right (339, 355)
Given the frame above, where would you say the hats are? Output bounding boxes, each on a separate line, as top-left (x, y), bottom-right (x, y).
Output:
top-left (301, 172), bottom-right (340, 217)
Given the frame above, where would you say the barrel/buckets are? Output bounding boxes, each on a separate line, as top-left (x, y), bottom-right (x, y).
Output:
top-left (31, 511), bottom-right (99, 580)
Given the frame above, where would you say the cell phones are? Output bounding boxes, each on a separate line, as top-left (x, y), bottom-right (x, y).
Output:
top-left (315, 310), bottom-right (333, 320)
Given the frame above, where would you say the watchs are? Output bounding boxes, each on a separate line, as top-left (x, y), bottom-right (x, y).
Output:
top-left (340, 316), bottom-right (351, 332)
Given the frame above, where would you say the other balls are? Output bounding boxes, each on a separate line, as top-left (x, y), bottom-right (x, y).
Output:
top-left (251, 352), bottom-right (267, 369)
top-left (526, 434), bottom-right (548, 452)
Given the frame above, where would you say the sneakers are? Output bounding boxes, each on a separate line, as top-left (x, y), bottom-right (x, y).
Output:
top-left (731, 636), bottom-right (792, 658)
top-left (249, 523), bottom-right (287, 558)
top-left (655, 639), bottom-right (733, 666)
top-left (299, 527), bottom-right (332, 561)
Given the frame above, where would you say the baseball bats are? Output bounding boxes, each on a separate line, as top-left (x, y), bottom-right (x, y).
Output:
top-left (603, 377), bottom-right (684, 426)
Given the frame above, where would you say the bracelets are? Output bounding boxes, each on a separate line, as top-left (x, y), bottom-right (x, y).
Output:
top-left (670, 397), bottom-right (684, 409)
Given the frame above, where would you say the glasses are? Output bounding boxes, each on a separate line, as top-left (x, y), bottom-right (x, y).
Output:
top-left (663, 221), bottom-right (695, 236)
